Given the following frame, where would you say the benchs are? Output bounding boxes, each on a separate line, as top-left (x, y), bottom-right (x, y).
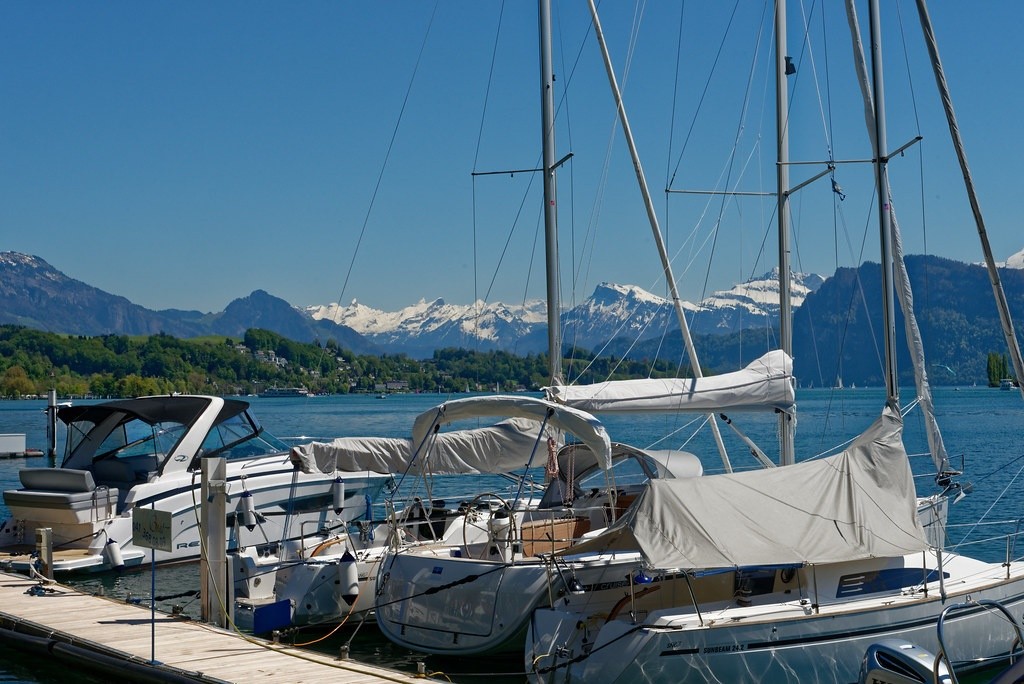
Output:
top-left (3, 467), bottom-right (118, 507)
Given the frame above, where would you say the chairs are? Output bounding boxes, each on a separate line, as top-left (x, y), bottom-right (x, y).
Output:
top-left (94, 460), bottom-right (137, 490)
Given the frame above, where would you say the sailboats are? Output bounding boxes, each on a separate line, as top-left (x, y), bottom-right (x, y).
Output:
top-left (1, 0), bottom-right (1024, 684)
